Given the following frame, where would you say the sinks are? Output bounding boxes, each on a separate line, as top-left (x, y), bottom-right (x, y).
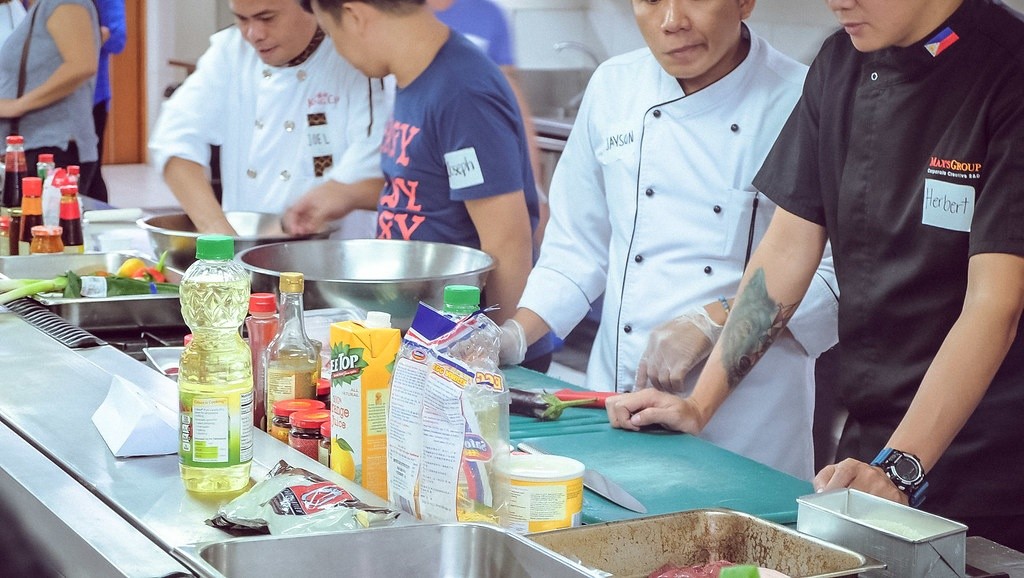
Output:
top-left (532, 113), bottom-right (574, 151)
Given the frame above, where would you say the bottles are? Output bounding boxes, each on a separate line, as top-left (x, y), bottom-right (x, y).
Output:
top-left (0, 134), bottom-right (84, 254)
top-left (245, 291), bottom-right (280, 429)
top-left (441, 284), bottom-right (481, 320)
top-left (278, 337), bottom-right (323, 382)
top-left (264, 270), bottom-right (319, 436)
top-left (179, 234), bottom-right (255, 494)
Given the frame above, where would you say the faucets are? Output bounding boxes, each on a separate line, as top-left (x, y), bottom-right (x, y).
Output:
top-left (555, 40), bottom-right (600, 68)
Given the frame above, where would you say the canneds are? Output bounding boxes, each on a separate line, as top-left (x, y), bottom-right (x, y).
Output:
top-left (0, 209), bottom-right (65, 257)
top-left (270, 379), bottom-right (333, 469)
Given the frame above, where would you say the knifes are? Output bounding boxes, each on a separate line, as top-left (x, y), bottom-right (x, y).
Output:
top-left (518, 441), bottom-right (648, 514)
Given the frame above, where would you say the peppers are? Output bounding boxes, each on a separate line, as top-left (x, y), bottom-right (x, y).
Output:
top-left (116, 250), bottom-right (167, 282)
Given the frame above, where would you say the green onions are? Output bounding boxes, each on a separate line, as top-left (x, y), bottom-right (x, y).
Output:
top-left (0, 270), bottom-right (180, 306)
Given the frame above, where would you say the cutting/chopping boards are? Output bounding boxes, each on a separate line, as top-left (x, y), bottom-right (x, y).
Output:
top-left (498, 365), bottom-right (815, 524)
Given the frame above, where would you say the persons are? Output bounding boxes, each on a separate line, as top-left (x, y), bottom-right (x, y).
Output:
top-left (147, 0), bottom-right (557, 376)
top-left (451, 0), bottom-right (838, 487)
top-left (0, 0), bottom-right (127, 203)
top-left (605, 0), bottom-right (1024, 523)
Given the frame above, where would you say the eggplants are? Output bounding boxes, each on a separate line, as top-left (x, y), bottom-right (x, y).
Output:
top-left (508, 389), bottom-right (597, 420)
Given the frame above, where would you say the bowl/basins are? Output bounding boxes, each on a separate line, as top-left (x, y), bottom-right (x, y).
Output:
top-left (134, 208), bottom-right (342, 275)
top-left (233, 238), bottom-right (501, 338)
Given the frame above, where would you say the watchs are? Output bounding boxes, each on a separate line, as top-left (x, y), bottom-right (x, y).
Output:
top-left (870, 444), bottom-right (930, 509)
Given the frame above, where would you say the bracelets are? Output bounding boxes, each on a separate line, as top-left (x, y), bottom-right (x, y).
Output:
top-left (718, 296), bottom-right (731, 316)
